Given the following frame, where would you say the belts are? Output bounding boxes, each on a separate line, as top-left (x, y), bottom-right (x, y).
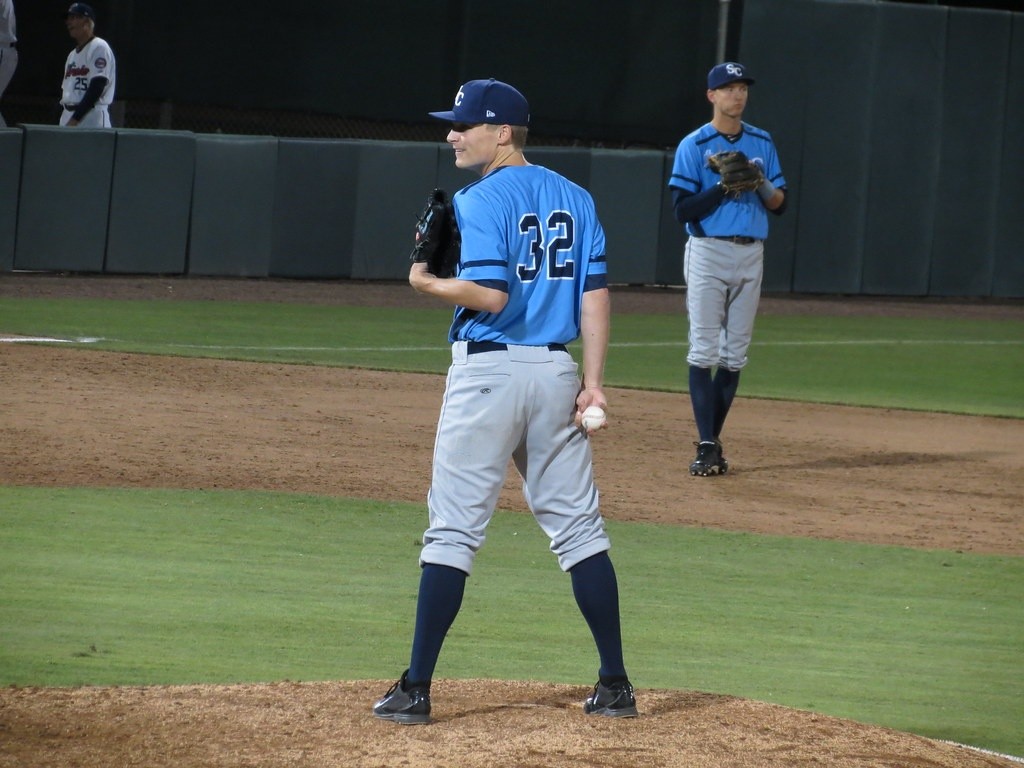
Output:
top-left (716, 236), bottom-right (756, 245)
top-left (467, 340), bottom-right (568, 354)
top-left (65, 102), bottom-right (95, 113)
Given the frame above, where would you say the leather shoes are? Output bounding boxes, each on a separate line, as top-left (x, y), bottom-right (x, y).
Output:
top-left (373, 668), bottom-right (431, 725)
top-left (584, 680), bottom-right (638, 718)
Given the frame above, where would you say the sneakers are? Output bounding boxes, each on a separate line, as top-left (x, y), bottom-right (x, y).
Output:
top-left (689, 438), bottom-right (728, 477)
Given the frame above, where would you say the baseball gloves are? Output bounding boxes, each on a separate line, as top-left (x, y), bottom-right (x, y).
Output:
top-left (409, 188), bottom-right (461, 278)
top-left (709, 150), bottom-right (764, 193)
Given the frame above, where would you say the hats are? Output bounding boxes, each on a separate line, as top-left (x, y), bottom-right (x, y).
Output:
top-left (67, 2), bottom-right (97, 24)
top-left (707, 62), bottom-right (756, 90)
top-left (427, 78), bottom-right (531, 126)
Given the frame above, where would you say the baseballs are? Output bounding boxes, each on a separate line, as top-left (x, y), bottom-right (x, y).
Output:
top-left (581, 405), bottom-right (606, 430)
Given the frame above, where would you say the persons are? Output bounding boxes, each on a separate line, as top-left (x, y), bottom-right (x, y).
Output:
top-left (668, 61), bottom-right (787, 477)
top-left (0, 0), bottom-right (18, 130)
top-left (58, 3), bottom-right (116, 128)
top-left (373, 78), bottom-right (639, 725)
